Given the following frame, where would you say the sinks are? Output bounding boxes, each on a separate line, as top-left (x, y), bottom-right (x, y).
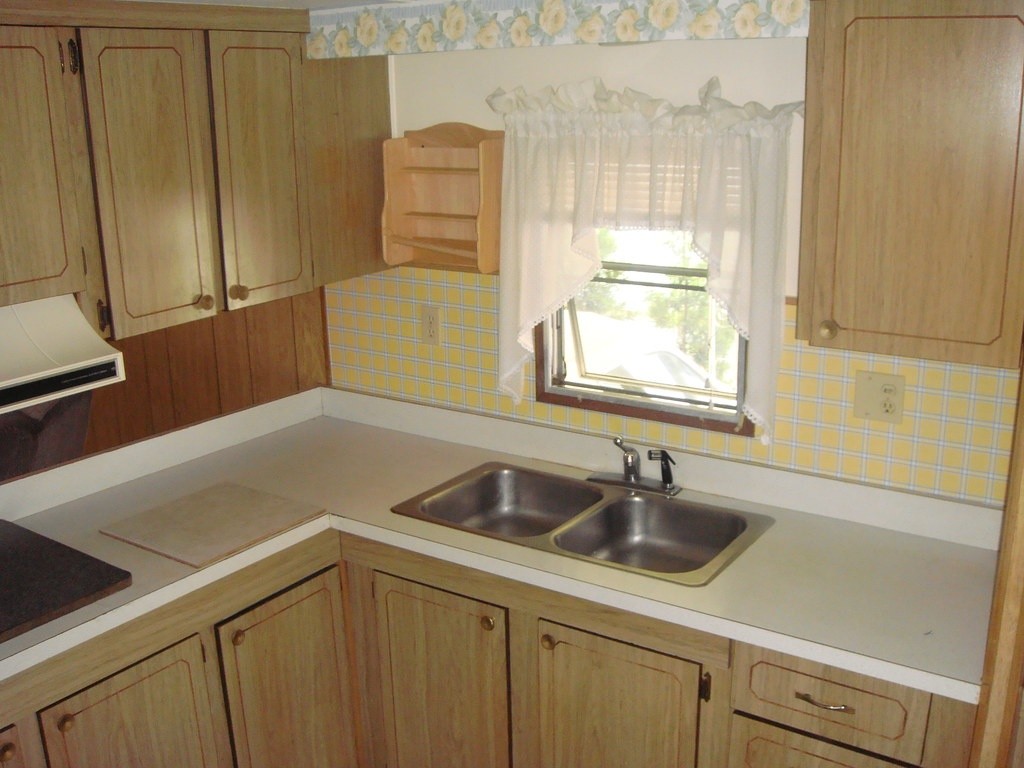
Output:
top-left (387, 458), bottom-right (610, 544)
top-left (541, 487), bottom-right (776, 587)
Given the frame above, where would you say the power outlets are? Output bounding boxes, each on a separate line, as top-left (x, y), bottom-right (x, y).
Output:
top-left (853, 370), bottom-right (904, 425)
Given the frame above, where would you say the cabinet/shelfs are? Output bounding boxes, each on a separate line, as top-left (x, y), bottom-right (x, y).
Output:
top-left (380, 120), bottom-right (505, 274)
top-left (796, 0), bottom-right (1024, 370)
top-left (0, 532), bottom-right (978, 768)
top-left (76, 25), bottom-right (316, 342)
top-left (0, 25), bottom-right (89, 309)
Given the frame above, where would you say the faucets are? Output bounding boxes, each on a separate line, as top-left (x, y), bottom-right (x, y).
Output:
top-left (612, 436), bottom-right (640, 480)
